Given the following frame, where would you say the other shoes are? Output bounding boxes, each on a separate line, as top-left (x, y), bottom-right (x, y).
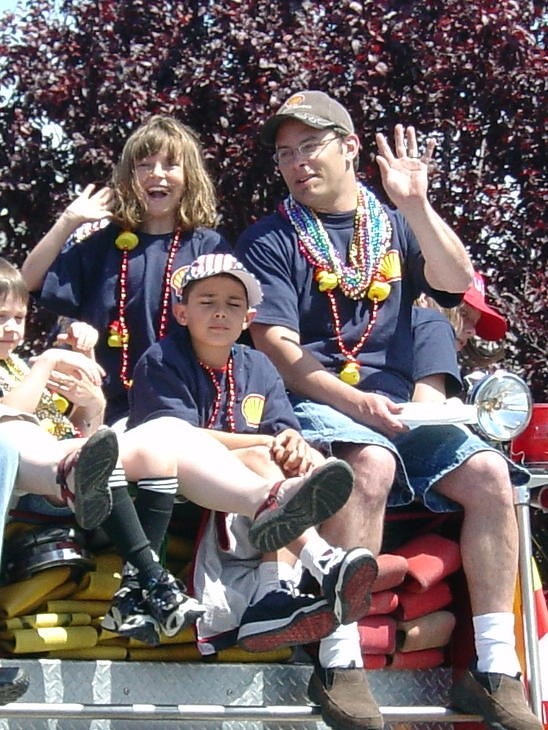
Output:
top-left (0, 664), bottom-right (30, 705)
top-left (449, 665), bottom-right (544, 730)
top-left (308, 659), bottom-right (385, 730)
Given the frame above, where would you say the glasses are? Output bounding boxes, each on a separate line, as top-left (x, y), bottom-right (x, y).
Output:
top-left (273, 136), bottom-right (343, 165)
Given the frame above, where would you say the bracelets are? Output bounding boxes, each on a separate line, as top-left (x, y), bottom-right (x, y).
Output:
top-left (80, 400), bottom-right (107, 428)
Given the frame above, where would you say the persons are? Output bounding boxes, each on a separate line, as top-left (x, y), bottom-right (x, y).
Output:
top-left (0, 115), bottom-right (378, 706)
top-left (235, 89), bottom-right (544, 730)
top-left (414, 270), bottom-right (508, 351)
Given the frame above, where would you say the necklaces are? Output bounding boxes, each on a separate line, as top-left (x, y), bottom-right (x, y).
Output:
top-left (199, 358), bottom-right (236, 433)
top-left (278, 181), bottom-right (392, 384)
top-left (117, 219), bottom-right (180, 390)
top-left (0, 357), bottom-right (79, 437)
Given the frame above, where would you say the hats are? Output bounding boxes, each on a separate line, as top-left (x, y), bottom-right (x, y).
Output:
top-left (182, 253), bottom-right (264, 308)
top-left (464, 272), bottom-right (508, 342)
top-left (262, 91), bottom-right (355, 136)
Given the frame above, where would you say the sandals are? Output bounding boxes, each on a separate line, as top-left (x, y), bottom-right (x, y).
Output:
top-left (57, 426), bottom-right (118, 529)
top-left (248, 456), bottom-right (354, 552)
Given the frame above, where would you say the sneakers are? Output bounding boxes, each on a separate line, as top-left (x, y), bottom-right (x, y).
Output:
top-left (101, 587), bottom-right (160, 645)
top-left (236, 578), bottom-right (340, 652)
top-left (140, 569), bottom-right (206, 638)
top-left (313, 546), bottom-right (378, 626)
top-left (198, 618), bottom-right (238, 654)
